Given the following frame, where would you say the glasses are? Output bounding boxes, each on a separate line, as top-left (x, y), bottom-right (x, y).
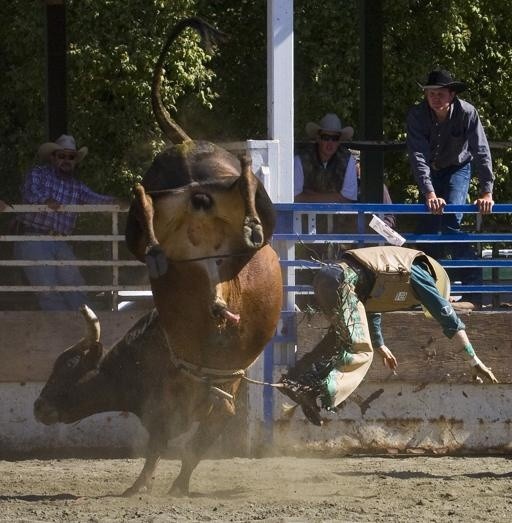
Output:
top-left (56, 153), bottom-right (75, 161)
top-left (320, 134), bottom-right (340, 142)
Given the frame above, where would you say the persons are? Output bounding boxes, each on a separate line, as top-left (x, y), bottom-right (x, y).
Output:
top-left (405, 68), bottom-right (498, 309)
top-left (277, 244), bottom-right (500, 427)
top-left (349, 148), bottom-right (398, 249)
top-left (12, 134), bottom-right (131, 311)
top-left (294, 112), bottom-right (359, 311)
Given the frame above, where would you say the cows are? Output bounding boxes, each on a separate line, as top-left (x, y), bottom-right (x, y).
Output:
top-left (33, 16), bottom-right (284, 498)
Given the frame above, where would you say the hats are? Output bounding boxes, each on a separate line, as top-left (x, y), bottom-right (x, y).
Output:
top-left (304, 113), bottom-right (354, 142)
top-left (37, 135), bottom-right (89, 164)
top-left (415, 70), bottom-right (467, 95)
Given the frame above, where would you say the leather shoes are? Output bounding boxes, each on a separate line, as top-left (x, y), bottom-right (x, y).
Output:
top-left (286, 380), bottom-right (324, 426)
top-left (277, 376), bottom-right (295, 405)
